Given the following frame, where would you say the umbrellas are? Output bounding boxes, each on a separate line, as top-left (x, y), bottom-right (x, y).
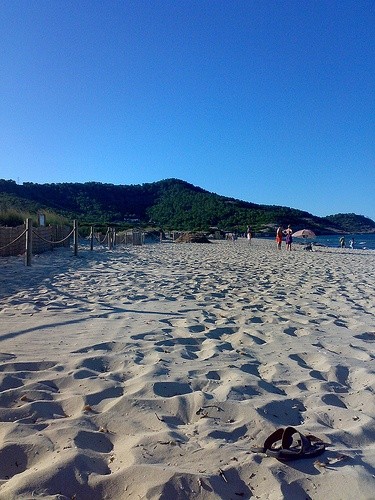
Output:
top-left (292, 229), bottom-right (317, 245)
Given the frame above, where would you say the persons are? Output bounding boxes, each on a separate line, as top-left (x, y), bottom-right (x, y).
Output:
top-left (232, 231), bottom-right (237, 244)
top-left (339, 235), bottom-right (344, 248)
top-left (283, 225), bottom-right (293, 252)
top-left (349, 237), bottom-right (354, 249)
top-left (246, 225), bottom-right (252, 247)
top-left (276, 227), bottom-right (282, 250)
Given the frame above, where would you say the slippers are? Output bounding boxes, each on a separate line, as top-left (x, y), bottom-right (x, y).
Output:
top-left (265, 427), bottom-right (325, 461)
